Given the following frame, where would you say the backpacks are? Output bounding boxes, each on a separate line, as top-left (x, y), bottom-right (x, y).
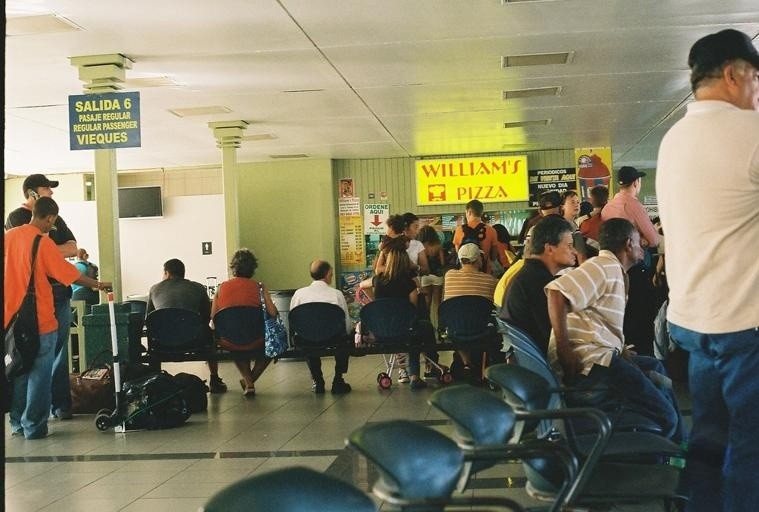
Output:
top-left (455, 223), bottom-right (487, 272)
top-left (96, 371), bottom-right (210, 432)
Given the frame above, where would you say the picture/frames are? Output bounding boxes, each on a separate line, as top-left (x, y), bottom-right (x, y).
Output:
top-left (338, 178), bottom-right (355, 198)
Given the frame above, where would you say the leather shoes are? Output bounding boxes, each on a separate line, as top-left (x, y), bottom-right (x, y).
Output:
top-left (311, 378), bottom-right (325, 393)
top-left (331, 377), bottom-right (351, 394)
top-left (240, 379), bottom-right (246, 389)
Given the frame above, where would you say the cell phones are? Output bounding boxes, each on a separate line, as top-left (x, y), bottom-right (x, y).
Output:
top-left (30, 191), bottom-right (38, 199)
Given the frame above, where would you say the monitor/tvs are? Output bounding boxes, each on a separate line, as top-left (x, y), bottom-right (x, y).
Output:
top-left (117, 185), bottom-right (163, 219)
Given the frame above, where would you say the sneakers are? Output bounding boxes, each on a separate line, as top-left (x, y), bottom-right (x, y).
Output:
top-left (210, 375), bottom-right (227, 393)
top-left (410, 378), bottom-right (428, 390)
top-left (51, 409), bottom-right (72, 420)
top-left (398, 367), bottom-right (410, 383)
top-left (423, 364), bottom-right (449, 379)
top-left (242, 388), bottom-right (255, 396)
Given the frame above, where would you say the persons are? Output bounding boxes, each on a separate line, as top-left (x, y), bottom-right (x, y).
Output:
top-left (358, 211), bottom-right (449, 388)
top-left (652, 27), bottom-right (759, 512)
top-left (491, 167), bottom-right (663, 366)
top-left (210, 246), bottom-right (279, 396)
top-left (444, 198), bottom-right (516, 375)
top-left (146, 258), bottom-right (227, 394)
top-left (6, 196), bottom-right (113, 440)
top-left (69, 246), bottom-right (93, 306)
top-left (5, 173), bottom-right (79, 419)
top-left (543, 214), bottom-right (680, 444)
top-left (288, 259), bottom-right (352, 393)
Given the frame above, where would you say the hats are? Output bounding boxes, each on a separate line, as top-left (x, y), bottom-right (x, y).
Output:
top-left (457, 242), bottom-right (484, 265)
top-left (687, 29), bottom-right (759, 68)
top-left (537, 190), bottom-right (563, 210)
top-left (617, 166), bottom-right (646, 185)
top-left (22, 174), bottom-right (59, 192)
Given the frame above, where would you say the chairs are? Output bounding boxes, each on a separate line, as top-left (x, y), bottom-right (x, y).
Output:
top-left (138, 291), bottom-right (496, 374)
top-left (203, 317), bottom-right (695, 512)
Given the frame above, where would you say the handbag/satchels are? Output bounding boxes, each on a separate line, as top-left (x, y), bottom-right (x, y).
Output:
top-left (70, 350), bottom-right (114, 414)
top-left (1, 294), bottom-right (43, 384)
top-left (257, 281), bottom-right (288, 359)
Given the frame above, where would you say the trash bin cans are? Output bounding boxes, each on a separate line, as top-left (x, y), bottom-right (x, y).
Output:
top-left (267, 288), bottom-right (298, 352)
